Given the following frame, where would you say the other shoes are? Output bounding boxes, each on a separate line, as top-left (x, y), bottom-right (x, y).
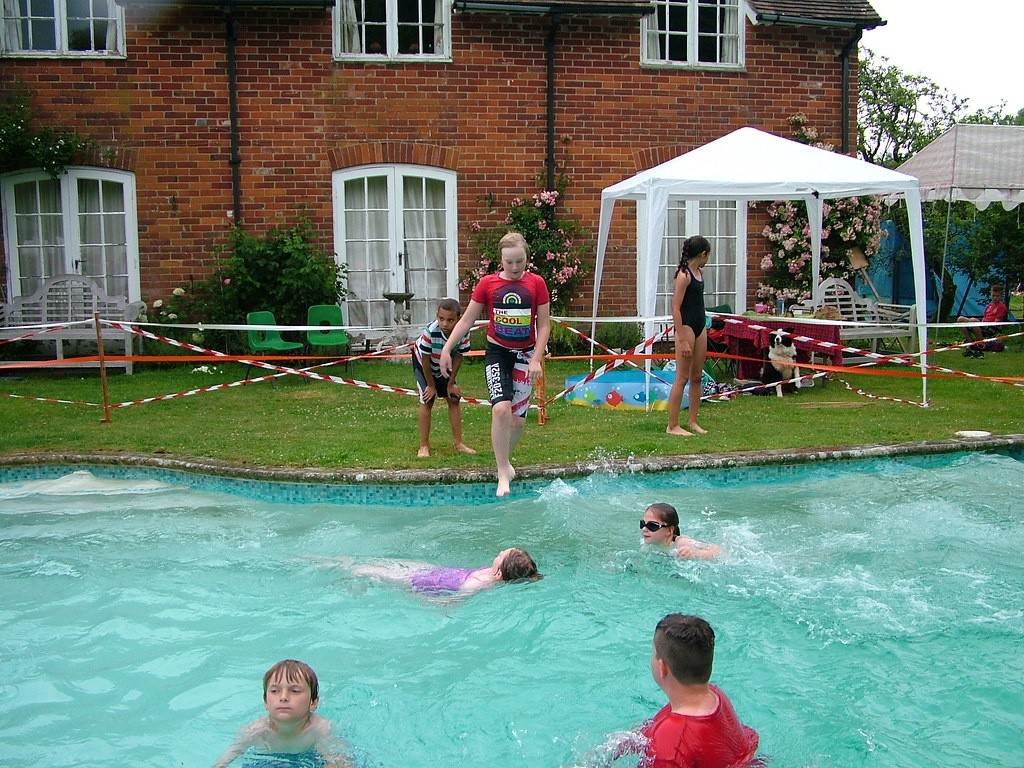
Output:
top-left (961, 348), bottom-right (985, 359)
top-left (702, 385), bottom-right (732, 403)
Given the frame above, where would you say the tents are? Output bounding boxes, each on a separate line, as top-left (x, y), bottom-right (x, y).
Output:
top-left (589, 125), bottom-right (931, 408)
top-left (884, 115), bottom-right (1024, 371)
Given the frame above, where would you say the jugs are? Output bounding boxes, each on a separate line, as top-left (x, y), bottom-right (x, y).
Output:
top-left (775, 299), bottom-right (787, 317)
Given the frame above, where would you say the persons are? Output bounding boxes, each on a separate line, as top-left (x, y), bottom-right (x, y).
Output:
top-left (664, 235), bottom-right (711, 437)
top-left (639, 502), bottom-right (732, 562)
top-left (411, 298), bottom-right (479, 457)
top-left (605, 611), bottom-right (766, 767)
top-left (209, 659), bottom-right (355, 767)
top-left (438, 231), bottom-right (552, 499)
top-left (957, 283), bottom-right (1007, 361)
top-left (353, 547), bottom-right (542, 607)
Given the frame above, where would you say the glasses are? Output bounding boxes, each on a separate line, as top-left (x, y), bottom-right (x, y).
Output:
top-left (639, 520), bottom-right (674, 532)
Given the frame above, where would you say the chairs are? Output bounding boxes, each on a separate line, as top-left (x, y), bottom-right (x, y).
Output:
top-left (241, 311), bottom-right (308, 388)
top-left (305, 305), bottom-right (356, 385)
top-left (795, 276), bottom-right (921, 364)
top-left (0, 274), bottom-right (148, 378)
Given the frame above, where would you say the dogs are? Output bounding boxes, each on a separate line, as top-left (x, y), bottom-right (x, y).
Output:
top-left (740, 327), bottom-right (802, 398)
